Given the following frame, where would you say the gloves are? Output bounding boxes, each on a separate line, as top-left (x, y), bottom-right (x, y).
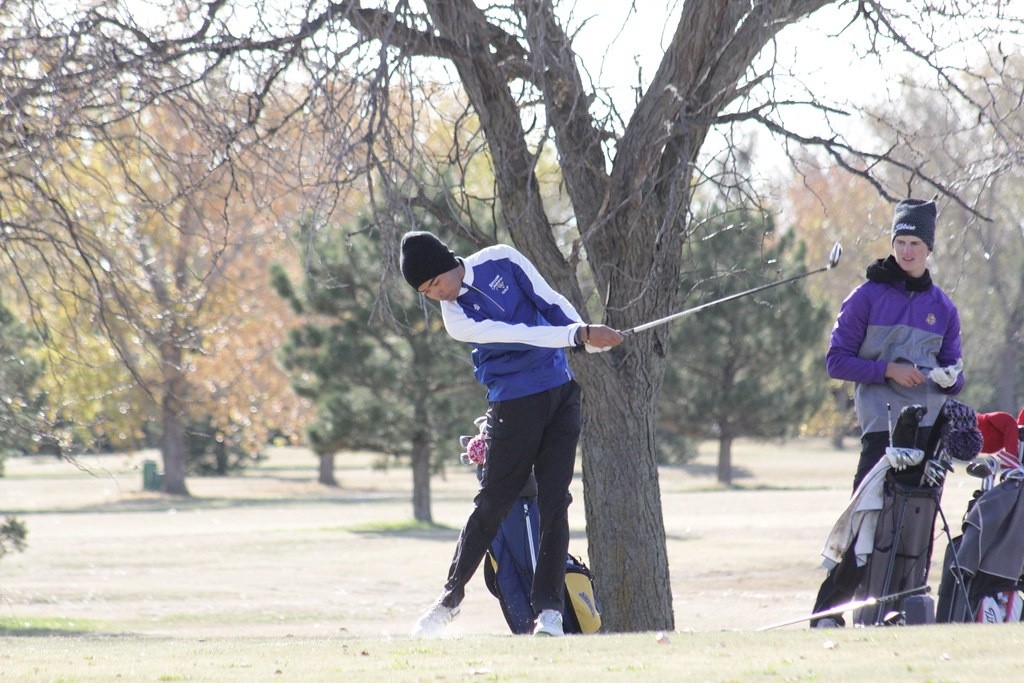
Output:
top-left (584, 342), bottom-right (612, 354)
top-left (930, 358), bottom-right (964, 387)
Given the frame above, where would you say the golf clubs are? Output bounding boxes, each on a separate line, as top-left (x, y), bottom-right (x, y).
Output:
top-left (619, 242), bottom-right (843, 337)
top-left (883, 399), bottom-right (1024, 490)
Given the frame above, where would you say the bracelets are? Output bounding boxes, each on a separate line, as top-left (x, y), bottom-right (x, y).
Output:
top-left (577, 325), bottom-right (584, 345)
top-left (585, 324), bottom-right (591, 344)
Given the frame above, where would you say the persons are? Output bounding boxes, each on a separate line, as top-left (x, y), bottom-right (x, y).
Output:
top-left (812, 198), bottom-right (966, 630)
top-left (398, 228), bottom-right (623, 637)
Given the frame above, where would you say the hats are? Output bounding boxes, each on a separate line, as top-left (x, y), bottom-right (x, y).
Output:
top-left (891, 199), bottom-right (937, 253)
top-left (399, 230), bottom-right (459, 292)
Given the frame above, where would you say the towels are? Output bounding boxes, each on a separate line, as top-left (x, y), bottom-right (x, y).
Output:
top-left (818, 446), bottom-right (1024, 587)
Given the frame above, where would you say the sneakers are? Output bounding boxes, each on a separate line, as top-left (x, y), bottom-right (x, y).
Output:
top-left (533, 610), bottom-right (564, 637)
top-left (413, 604), bottom-right (461, 638)
